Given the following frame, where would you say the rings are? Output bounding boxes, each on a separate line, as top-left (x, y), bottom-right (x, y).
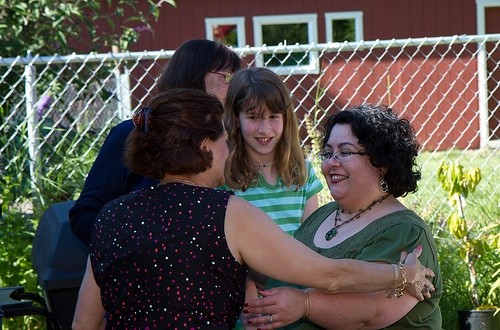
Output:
top-left (269, 316), bottom-right (273, 324)
top-left (423, 286), bottom-right (430, 296)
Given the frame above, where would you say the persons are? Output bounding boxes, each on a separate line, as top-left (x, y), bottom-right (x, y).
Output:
top-left (213, 66), bottom-right (324, 330)
top-left (68, 38), bottom-right (241, 247)
top-left (242, 103), bottom-right (444, 330)
top-left (71, 86), bottom-right (435, 330)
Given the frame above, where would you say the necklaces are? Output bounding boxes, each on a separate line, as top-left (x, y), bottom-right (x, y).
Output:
top-left (252, 160), bottom-right (278, 167)
top-left (325, 192), bottom-right (393, 241)
top-left (167, 176), bottom-right (210, 188)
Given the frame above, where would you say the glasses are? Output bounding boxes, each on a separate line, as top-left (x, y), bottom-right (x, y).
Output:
top-left (321, 149), bottom-right (368, 162)
top-left (209, 71), bottom-right (232, 83)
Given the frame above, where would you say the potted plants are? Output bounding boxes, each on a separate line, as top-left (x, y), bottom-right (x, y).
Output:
top-left (439, 157), bottom-right (500, 330)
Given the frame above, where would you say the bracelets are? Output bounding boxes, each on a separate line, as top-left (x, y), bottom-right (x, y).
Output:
top-left (302, 288), bottom-right (311, 318)
top-left (385, 262), bottom-right (408, 300)
